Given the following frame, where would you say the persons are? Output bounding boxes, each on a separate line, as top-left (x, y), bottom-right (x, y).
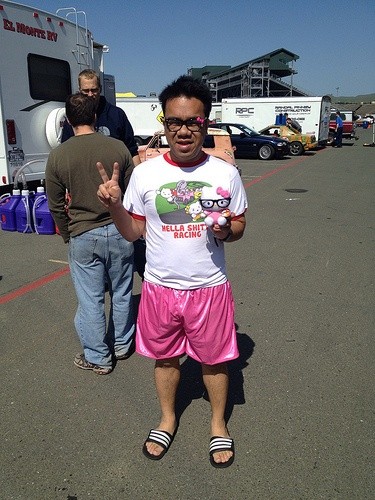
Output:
top-left (97, 75), bottom-right (248, 468)
top-left (45, 94), bottom-right (136, 375)
top-left (334, 111), bottom-right (343, 147)
top-left (60, 69), bottom-right (147, 282)
top-left (287, 118), bottom-right (301, 132)
top-left (273, 130), bottom-right (279, 137)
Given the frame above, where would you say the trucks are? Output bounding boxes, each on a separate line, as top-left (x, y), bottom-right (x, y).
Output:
top-left (0, 0), bottom-right (110, 188)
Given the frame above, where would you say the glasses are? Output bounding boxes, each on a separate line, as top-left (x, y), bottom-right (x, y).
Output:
top-left (165, 117), bottom-right (208, 132)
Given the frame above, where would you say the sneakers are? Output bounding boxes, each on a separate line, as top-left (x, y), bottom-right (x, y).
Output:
top-left (74, 352), bottom-right (111, 375)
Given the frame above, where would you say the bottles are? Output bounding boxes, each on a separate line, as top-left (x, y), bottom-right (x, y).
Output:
top-left (14, 189), bottom-right (34, 233)
top-left (0, 189), bottom-right (21, 231)
top-left (32, 186), bottom-right (55, 234)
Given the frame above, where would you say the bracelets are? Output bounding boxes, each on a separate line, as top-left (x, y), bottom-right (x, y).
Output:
top-left (219, 231), bottom-right (231, 241)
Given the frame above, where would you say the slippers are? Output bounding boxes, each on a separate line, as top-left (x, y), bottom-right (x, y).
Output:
top-left (143, 417), bottom-right (180, 461)
top-left (209, 426), bottom-right (235, 468)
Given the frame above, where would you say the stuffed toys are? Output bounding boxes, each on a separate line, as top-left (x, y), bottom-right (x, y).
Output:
top-left (199, 186), bottom-right (232, 226)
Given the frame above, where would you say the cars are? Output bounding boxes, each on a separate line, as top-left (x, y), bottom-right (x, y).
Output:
top-left (257, 125), bottom-right (319, 157)
top-left (203, 123), bottom-right (291, 160)
